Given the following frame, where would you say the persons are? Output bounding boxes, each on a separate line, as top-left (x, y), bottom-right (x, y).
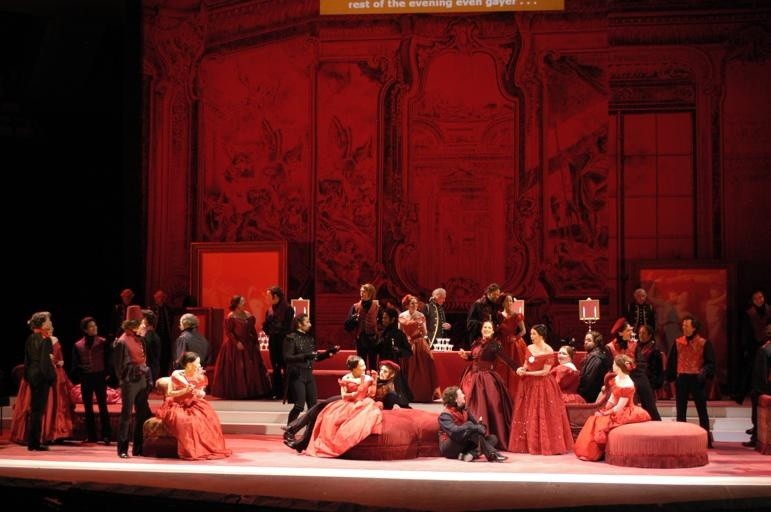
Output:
top-left (740, 289), bottom-right (771, 449)
top-left (283, 314), bottom-right (341, 425)
top-left (109, 288), bottom-right (172, 457)
top-left (174, 313), bottom-right (212, 370)
top-left (263, 287), bottom-right (295, 399)
top-left (282, 357), bottom-right (410, 458)
top-left (438, 387), bottom-right (508, 462)
top-left (508, 324), bottom-right (578, 454)
top-left (458, 284), bottom-right (526, 450)
top-left (74, 317), bottom-right (111, 445)
top-left (156, 352), bottom-right (232, 460)
top-left (574, 319), bottom-right (663, 461)
top-left (344, 283), bottom-right (451, 402)
top-left (666, 316), bottom-right (716, 447)
top-left (9, 312), bottom-right (77, 451)
top-left (628, 289), bottom-right (655, 339)
top-left (212, 296), bottom-right (272, 399)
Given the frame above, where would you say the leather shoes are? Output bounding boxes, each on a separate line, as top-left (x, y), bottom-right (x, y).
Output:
top-left (281, 426), bottom-right (303, 454)
top-left (28, 445), bottom-right (47, 450)
top-left (488, 453), bottom-right (508, 461)
top-left (118, 453), bottom-right (128, 458)
top-left (458, 452), bottom-right (473, 462)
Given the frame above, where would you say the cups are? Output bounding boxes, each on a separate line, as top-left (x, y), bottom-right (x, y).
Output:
top-left (433, 338), bottom-right (455, 351)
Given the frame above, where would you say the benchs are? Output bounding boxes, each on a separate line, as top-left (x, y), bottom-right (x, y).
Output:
top-left (257, 345), bottom-right (362, 399)
top-left (606, 419), bottom-right (709, 469)
top-left (339, 409), bottom-right (442, 459)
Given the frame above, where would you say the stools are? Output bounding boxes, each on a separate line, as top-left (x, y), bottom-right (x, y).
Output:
top-left (142, 416), bottom-right (178, 457)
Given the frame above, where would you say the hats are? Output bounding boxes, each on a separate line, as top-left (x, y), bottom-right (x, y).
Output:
top-left (126, 305), bottom-right (144, 321)
top-left (378, 360), bottom-right (400, 372)
top-left (610, 316), bottom-right (626, 335)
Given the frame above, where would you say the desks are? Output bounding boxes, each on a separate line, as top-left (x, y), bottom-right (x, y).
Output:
top-left (430, 348), bottom-right (589, 402)
